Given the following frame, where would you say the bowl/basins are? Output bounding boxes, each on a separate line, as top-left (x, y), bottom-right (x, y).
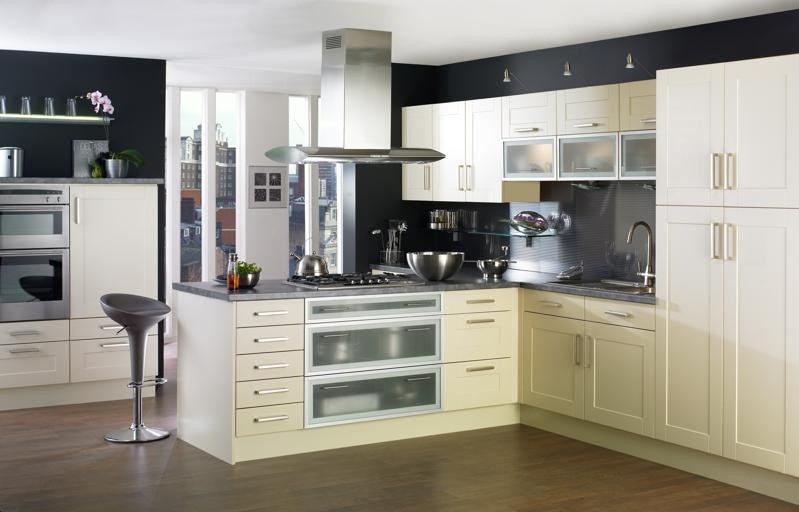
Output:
top-left (406, 251), bottom-right (465, 283)
top-left (240, 271), bottom-right (262, 290)
top-left (477, 259), bottom-right (509, 279)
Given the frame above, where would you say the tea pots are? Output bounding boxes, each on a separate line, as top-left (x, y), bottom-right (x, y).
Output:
top-left (289, 251), bottom-right (332, 279)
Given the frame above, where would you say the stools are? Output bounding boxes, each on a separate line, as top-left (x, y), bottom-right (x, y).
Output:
top-left (100, 290), bottom-right (171, 441)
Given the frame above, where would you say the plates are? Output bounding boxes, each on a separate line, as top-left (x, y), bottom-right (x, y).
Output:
top-left (213, 278), bottom-right (227, 285)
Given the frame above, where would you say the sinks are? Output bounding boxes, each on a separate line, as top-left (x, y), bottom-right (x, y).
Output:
top-left (573, 282), bottom-right (645, 294)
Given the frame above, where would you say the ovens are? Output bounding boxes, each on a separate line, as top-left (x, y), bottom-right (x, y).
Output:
top-left (0, 249), bottom-right (70, 323)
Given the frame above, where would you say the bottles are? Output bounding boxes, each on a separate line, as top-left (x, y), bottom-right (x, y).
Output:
top-left (227, 253), bottom-right (240, 290)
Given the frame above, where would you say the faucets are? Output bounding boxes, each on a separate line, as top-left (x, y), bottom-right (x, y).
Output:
top-left (626, 220), bottom-right (655, 286)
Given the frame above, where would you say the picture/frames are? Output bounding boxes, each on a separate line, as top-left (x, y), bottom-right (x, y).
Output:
top-left (247, 165), bottom-right (288, 210)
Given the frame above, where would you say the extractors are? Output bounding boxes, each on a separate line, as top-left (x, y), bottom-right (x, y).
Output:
top-left (263, 27), bottom-right (447, 166)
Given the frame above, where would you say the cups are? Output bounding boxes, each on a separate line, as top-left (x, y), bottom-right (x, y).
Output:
top-left (66, 98), bottom-right (78, 116)
top-left (43, 96), bottom-right (56, 114)
top-left (1, 95), bottom-right (7, 116)
top-left (19, 95), bottom-right (32, 114)
top-left (430, 209), bottom-right (477, 231)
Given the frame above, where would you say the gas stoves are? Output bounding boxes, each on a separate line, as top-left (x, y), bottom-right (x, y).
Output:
top-left (288, 271), bottom-right (413, 287)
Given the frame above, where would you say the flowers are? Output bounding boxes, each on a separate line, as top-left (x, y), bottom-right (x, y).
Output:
top-left (71, 92), bottom-right (148, 179)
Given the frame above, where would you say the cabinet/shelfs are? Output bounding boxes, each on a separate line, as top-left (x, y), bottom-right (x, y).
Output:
top-left (520, 288), bottom-right (657, 439)
top-left (657, 48), bottom-right (797, 207)
top-left (441, 287), bottom-right (520, 412)
top-left (70, 179), bottom-right (164, 319)
top-left (401, 105), bottom-right (429, 201)
top-left (657, 206), bottom-right (797, 485)
top-left (430, 96), bottom-right (540, 203)
top-left (305, 292), bottom-right (440, 430)
top-left (1, 320), bottom-right (159, 410)
top-left (173, 291), bottom-right (306, 438)
top-left (500, 78), bottom-right (657, 181)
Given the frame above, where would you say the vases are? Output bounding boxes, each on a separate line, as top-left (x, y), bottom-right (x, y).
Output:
top-left (100, 156), bottom-right (132, 177)
